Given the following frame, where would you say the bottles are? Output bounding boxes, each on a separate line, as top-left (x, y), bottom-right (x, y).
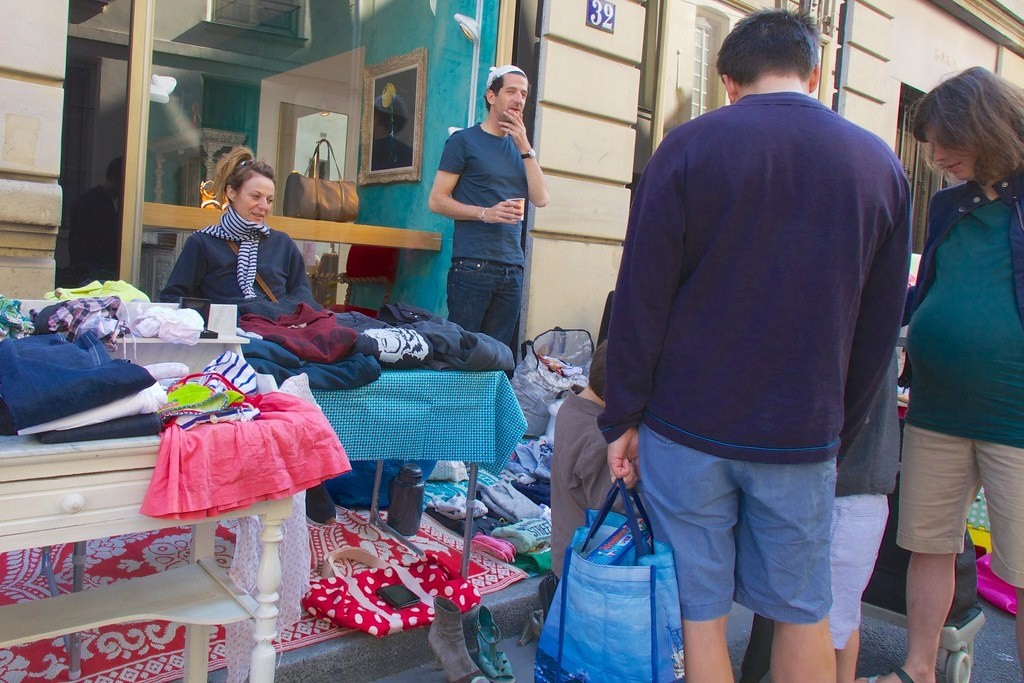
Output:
top-left (387, 465), bottom-right (424, 536)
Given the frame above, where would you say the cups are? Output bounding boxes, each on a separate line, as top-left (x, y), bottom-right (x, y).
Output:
top-left (507, 198), bottom-right (525, 221)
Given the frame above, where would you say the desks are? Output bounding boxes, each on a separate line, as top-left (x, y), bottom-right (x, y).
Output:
top-left (0, 300), bottom-right (293, 683)
top-left (312, 367), bottom-right (528, 580)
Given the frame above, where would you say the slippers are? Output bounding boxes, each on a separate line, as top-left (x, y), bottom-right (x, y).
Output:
top-left (858, 668), bottom-right (915, 683)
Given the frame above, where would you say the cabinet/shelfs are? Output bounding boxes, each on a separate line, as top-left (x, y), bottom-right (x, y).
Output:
top-left (139, 128), bottom-right (248, 302)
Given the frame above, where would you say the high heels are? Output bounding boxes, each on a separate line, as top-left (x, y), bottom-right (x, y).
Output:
top-left (472, 605), bottom-right (512, 683)
top-left (428, 595), bottom-right (489, 683)
top-left (520, 611), bottom-right (546, 646)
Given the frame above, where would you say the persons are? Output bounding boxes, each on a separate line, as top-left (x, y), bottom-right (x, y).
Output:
top-left (371, 94), bottom-right (414, 171)
top-left (429, 65), bottom-right (549, 348)
top-left (550, 9), bottom-right (1024, 682)
top-left (162, 144), bottom-right (337, 527)
top-left (69, 157), bottom-right (124, 287)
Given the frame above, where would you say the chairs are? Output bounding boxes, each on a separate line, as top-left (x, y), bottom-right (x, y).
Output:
top-left (318, 243), bottom-right (400, 320)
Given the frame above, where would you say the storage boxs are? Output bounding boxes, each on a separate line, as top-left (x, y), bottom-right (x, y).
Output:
top-left (586, 518), bottom-right (652, 566)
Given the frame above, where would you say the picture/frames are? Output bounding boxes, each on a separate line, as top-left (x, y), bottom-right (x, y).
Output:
top-left (453, 12), bottom-right (479, 43)
top-left (358, 48), bottom-right (427, 185)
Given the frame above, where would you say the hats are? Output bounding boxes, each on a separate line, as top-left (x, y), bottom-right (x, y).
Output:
top-left (485, 62), bottom-right (526, 90)
top-left (376, 93), bottom-right (406, 117)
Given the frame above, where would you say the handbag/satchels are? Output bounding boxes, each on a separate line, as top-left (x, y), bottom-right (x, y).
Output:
top-left (536, 476), bottom-right (686, 683)
top-left (512, 326), bottom-right (594, 436)
top-left (282, 139), bottom-right (358, 221)
top-left (302, 547), bottom-right (481, 639)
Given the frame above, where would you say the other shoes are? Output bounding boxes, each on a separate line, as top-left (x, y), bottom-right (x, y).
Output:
top-left (307, 485), bottom-right (336, 526)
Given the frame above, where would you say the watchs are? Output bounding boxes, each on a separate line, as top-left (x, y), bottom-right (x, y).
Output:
top-left (521, 149), bottom-right (535, 159)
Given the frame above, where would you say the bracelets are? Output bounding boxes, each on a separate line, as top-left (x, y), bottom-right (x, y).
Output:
top-left (482, 208), bottom-right (489, 223)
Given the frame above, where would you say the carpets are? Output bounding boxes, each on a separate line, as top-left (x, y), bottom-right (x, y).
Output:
top-left (0, 509), bottom-right (527, 683)
